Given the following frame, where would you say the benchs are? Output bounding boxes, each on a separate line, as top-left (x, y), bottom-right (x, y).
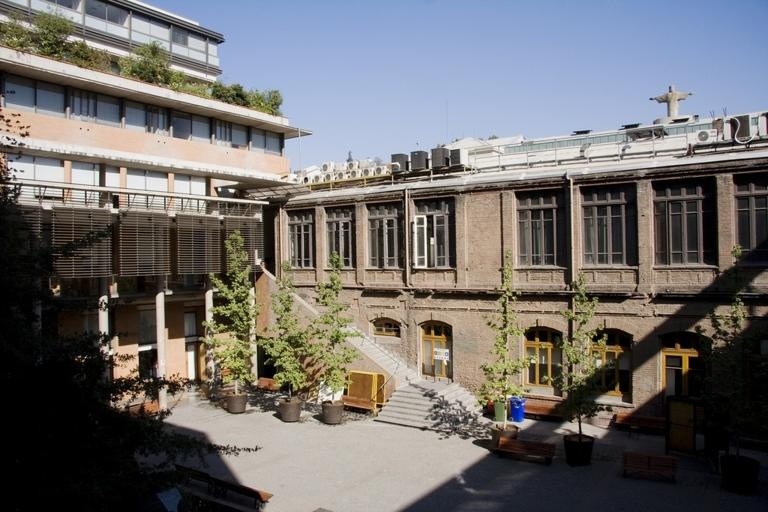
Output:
top-left (174, 464), bottom-right (274, 512)
top-left (126, 399), bottom-right (159, 418)
top-left (620, 452), bottom-right (684, 483)
top-left (490, 435), bottom-right (556, 467)
top-left (523, 404), bottom-right (568, 421)
top-left (611, 412), bottom-right (666, 435)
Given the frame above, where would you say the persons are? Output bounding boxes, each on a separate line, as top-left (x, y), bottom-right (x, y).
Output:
top-left (650, 84), bottom-right (694, 116)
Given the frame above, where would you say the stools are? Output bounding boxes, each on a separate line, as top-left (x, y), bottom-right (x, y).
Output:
top-left (628, 425), bottom-right (641, 442)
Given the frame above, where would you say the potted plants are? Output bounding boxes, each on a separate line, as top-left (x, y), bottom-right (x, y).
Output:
top-left (556, 269), bottom-right (609, 466)
top-left (708, 240), bottom-right (768, 497)
top-left (479, 248), bottom-right (539, 423)
top-left (203, 230), bottom-right (365, 425)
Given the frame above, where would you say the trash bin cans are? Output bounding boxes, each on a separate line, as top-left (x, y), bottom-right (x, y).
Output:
top-left (508, 397), bottom-right (526, 422)
top-left (493, 400), bottom-right (508, 422)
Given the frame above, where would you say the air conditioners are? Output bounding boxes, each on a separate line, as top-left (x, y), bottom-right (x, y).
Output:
top-left (691, 113), bottom-right (768, 144)
top-left (300, 159), bottom-right (389, 185)
